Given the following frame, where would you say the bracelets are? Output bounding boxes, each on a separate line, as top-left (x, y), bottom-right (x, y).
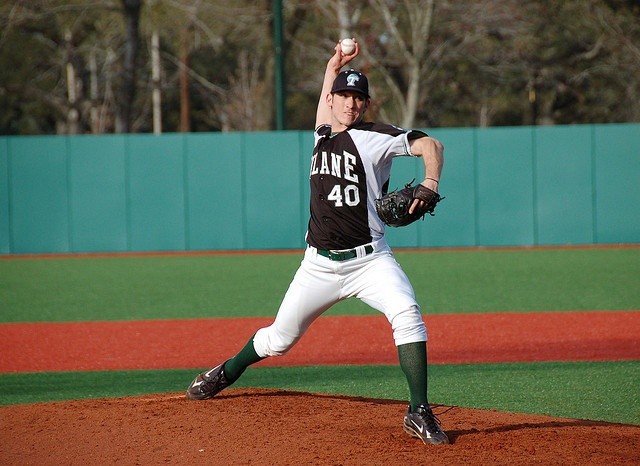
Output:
top-left (424, 177), bottom-right (440, 185)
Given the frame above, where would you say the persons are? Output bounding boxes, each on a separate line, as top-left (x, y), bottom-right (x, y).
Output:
top-left (186, 37), bottom-right (451, 446)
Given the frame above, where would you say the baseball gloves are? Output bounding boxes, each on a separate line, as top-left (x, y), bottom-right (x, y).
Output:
top-left (374, 177), bottom-right (445, 227)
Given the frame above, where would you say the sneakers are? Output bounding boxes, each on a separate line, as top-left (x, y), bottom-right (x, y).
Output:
top-left (185, 356), bottom-right (247, 402)
top-left (402, 401), bottom-right (449, 447)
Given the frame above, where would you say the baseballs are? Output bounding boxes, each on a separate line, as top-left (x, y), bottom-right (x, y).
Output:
top-left (340, 37), bottom-right (356, 56)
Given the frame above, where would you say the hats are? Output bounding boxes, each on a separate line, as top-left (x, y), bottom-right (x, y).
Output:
top-left (329, 68), bottom-right (371, 101)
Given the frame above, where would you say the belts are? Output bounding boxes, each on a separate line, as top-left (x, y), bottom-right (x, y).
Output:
top-left (308, 243), bottom-right (374, 263)
